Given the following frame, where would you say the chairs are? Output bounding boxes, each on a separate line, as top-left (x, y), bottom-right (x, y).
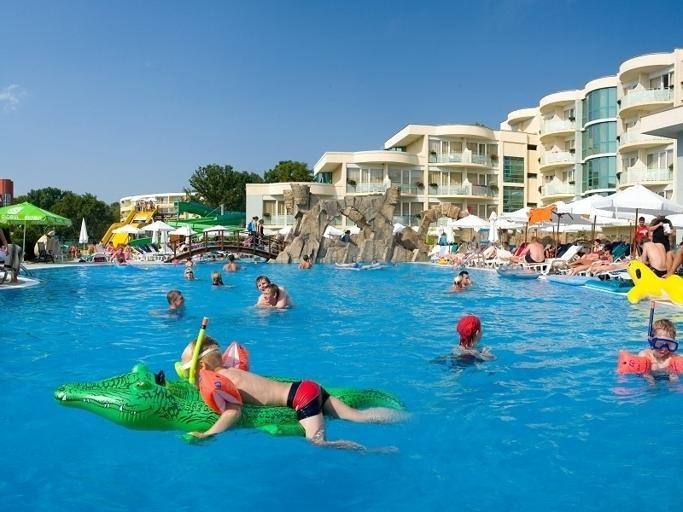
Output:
top-left (452, 242), bottom-right (671, 292)
top-left (130, 244), bottom-right (174, 261)
top-left (37, 242), bottom-right (54, 264)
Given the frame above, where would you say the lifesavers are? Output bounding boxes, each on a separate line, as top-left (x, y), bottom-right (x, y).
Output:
top-left (197, 366), bottom-right (245, 414)
top-left (627, 259), bottom-right (683, 306)
top-left (53, 360), bottom-right (411, 436)
top-left (221, 341), bottom-right (249, 372)
top-left (669, 356), bottom-right (683, 374)
top-left (618, 349), bottom-right (652, 372)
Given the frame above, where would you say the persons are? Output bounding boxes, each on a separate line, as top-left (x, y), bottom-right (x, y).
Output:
top-left (263, 284), bottom-right (289, 310)
top-left (0, 227), bottom-right (9, 257)
top-left (340, 229), bottom-right (353, 243)
top-left (66, 240), bottom-right (135, 265)
top-left (299, 253), bottom-right (312, 269)
top-left (134, 200), bottom-right (155, 213)
top-left (637, 318), bottom-right (680, 388)
top-left (438, 214), bottom-right (683, 276)
top-left (180, 335), bottom-right (411, 454)
top-left (197, 252), bottom-right (228, 261)
top-left (223, 254), bottom-right (239, 272)
top-left (451, 314), bottom-right (497, 362)
top-left (185, 268), bottom-right (193, 280)
top-left (335, 255), bottom-right (380, 270)
top-left (185, 256), bottom-right (194, 267)
top-left (448, 270), bottom-right (473, 291)
top-left (42, 235), bottom-right (51, 254)
top-left (167, 290), bottom-right (184, 311)
top-left (212, 272), bottom-right (223, 286)
top-left (257, 219), bottom-right (264, 245)
top-left (256, 275), bottom-right (291, 309)
top-left (247, 216), bottom-right (258, 244)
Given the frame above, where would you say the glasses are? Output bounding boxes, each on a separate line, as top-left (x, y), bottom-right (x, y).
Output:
top-left (651, 337), bottom-right (679, 353)
top-left (174, 349), bottom-right (218, 378)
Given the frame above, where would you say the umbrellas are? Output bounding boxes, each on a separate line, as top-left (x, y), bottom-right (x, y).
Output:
top-left (0, 200), bottom-right (72, 263)
top-left (111, 219), bottom-right (230, 254)
top-left (438, 181), bottom-right (683, 261)
top-left (79, 217), bottom-right (88, 252)
